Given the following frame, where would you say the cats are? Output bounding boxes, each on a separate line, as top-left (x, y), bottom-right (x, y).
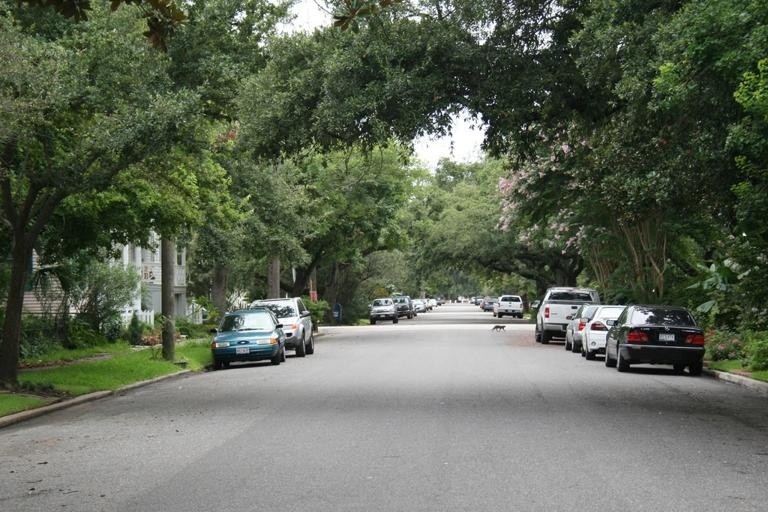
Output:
top-left (491, 325), bottom-right (506, 331)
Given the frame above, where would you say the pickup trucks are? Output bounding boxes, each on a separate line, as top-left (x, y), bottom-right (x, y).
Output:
top-left (531, 284), bottom-right (600, 343)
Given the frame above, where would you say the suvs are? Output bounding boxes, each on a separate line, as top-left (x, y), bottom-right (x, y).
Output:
top-left (249, 297), bottom-right (315, 357)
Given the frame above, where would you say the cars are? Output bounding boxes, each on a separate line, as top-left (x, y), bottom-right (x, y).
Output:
top-left (458, 295), bottom-right (526, 318)
top-left (212, 307), bottom-right (286, 369)
top-left (565, 303), bottom-right (706, 375)
top-left (367, 292), bottom-right (446, 325)
